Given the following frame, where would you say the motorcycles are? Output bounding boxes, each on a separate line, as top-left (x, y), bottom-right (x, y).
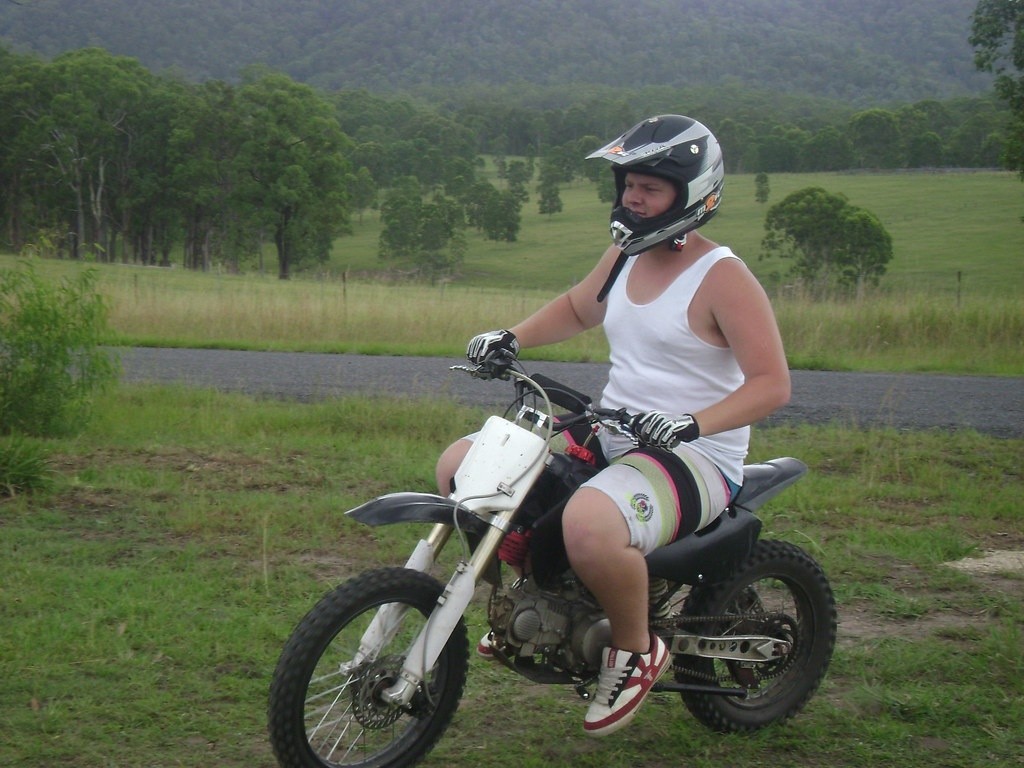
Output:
top-left (262, 349), bottom-right (839, 768)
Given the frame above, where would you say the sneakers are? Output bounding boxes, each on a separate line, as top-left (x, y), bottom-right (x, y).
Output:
top-left (582, 631), bottom-right (672, 738)
top-left (477, 630), bottom-right (508, 660)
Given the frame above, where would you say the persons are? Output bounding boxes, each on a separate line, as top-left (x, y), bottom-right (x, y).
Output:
top-left (436, 114), bottom-right (791, 738)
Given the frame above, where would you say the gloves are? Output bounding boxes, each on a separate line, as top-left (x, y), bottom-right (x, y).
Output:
top-left (466, 328), bottom-right (520, 367)
top-left (631, 411), bottom-right (700, 452)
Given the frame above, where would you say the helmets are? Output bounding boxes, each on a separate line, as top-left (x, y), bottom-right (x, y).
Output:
top-left (584, 114), bottom-right (724, 257)
